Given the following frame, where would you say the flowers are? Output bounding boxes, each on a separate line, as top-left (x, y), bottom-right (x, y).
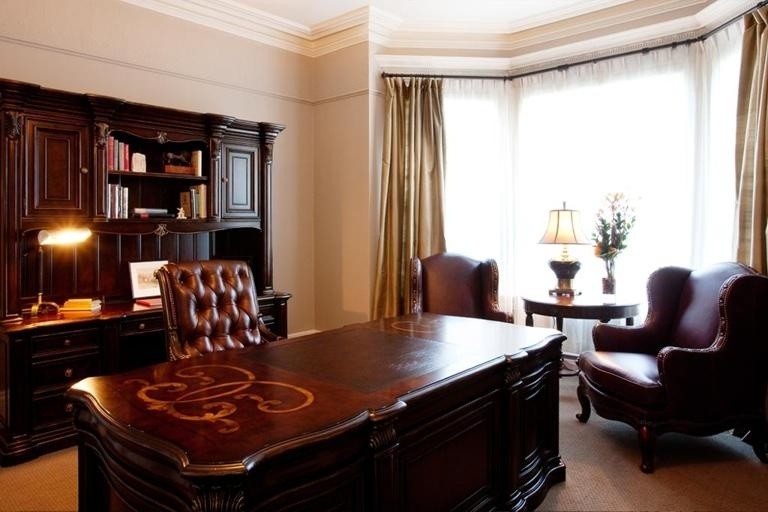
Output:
top-left (590, 191), bottom-right (636, 277)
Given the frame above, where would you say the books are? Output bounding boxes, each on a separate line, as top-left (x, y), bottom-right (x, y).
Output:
top-left (108, 183), bottom-right (129, 219)
top-left (179, 183), bottom-right (208, 220)
top-left (191, 150), bottom-right (202, 177)
top-left (130, 207), bottom-right (175, 218)
top-left (108, 135), bottom-right (130, 171)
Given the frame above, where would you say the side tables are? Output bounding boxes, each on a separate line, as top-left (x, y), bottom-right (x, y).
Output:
top-left (521, 297), bottom-right (640, 377)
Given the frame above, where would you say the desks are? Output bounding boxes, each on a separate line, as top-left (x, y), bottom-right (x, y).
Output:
top-left (64, 313), bottom-right (567, 512)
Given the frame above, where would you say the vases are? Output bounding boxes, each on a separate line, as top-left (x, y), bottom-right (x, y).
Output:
top-left (603, 275), bottom-right (617, 295)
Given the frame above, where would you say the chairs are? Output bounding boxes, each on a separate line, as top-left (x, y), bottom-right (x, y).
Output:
top-left (576, 262), bottom-right (768, 474)
top-left (409, 253), bottom-right (514, 323)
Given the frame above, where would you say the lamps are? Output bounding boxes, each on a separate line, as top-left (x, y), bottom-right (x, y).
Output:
top-left (538, 202), bottom-right (591, 297)
top-left (28, 229), bottom-right (92, 318)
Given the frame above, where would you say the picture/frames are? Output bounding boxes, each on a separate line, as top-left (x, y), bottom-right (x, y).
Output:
top-left (128, 259), bottom-right (169, 300)
